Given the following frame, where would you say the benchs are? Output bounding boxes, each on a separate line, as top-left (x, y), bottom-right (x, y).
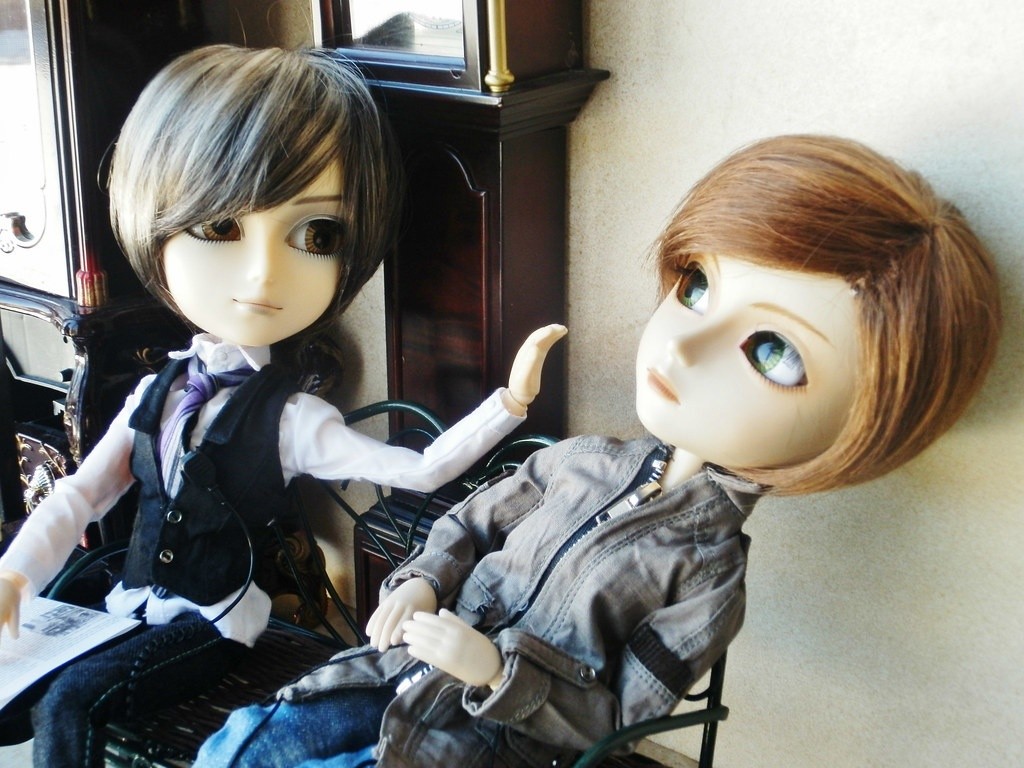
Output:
top-left (48, 398), bottom-right (752, 768)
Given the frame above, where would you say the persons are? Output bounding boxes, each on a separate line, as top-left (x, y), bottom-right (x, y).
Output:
top-left (0, 47), bottom-right (569, 768)
top-left (186, 134), bottom-right (1001, 768)
